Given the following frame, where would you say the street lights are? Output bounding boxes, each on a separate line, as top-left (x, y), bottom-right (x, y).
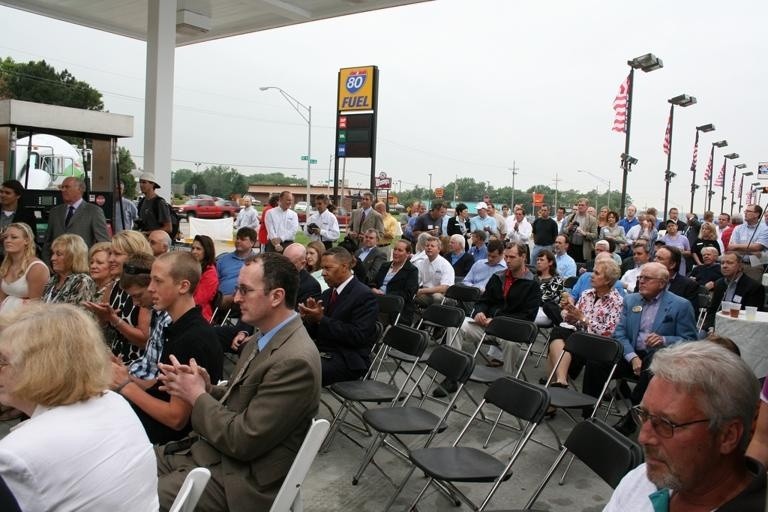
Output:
top-left (619, 53), bottom-right (665, 220)
top-left (195, 163), bottom-right (202, 194)
top-left (660, 197), bottom-right (685, 221)
top-left (664, 93), bottom-right (698, 222)
top-left (428, 173), bottom-right (432, 209)
top-left (397, 180), bottom-right (401, 204)
top-left (257, 84), bottom-right (313, 203)
top-left (707, 139), bottom-right (764, 216)
top-left (690, 123), bottom-right (717, 216)
top-left (577, 169), bottom-right (613, 212)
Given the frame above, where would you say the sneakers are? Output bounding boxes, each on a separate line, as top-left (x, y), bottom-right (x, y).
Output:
top-left (432, 378), bottom-right (458, 397)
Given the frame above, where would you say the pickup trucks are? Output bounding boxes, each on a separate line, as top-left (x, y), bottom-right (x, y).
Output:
top-left (173, 198), bottom-right (234, 220)
top-left (215, 201), bottom-right (242, 213)
top-left (191, 193), bottom-right (211, 199)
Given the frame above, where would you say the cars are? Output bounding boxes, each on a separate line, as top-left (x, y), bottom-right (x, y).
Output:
top-left (294, 200), bottom-right (312, 212)
top-left (307, 206), bottom-right (349, 227)
top-left (250, 197), bottom-right (261, 206)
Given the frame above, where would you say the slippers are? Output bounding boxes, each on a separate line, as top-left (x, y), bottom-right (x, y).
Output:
top-left (550, 382), bottom-right (569, 389)
top-left (545, 406), bottom-right (557, 416)
top-left (485, 359), bottom-right (504, 367)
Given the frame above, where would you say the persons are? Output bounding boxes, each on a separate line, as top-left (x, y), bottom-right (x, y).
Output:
top-left (1, 174), bottom-right (768, 512)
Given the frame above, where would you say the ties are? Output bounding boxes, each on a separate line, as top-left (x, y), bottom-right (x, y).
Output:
top-left (172, 341), bottom-right (259, 456)
top-left (65, 205), bottom-right (74, 228)
top-left (359, 211), bottom-right (365, 235)
top-left (327, 289), bottom-right (338, 318)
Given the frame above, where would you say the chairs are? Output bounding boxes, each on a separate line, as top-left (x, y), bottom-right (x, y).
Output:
top-left (515, 417), bottom-right (650, 511)
top-left (161, 463), bottom-right (214, 512)
top-left (438, 260), bottom-right (711, 367)
top-left (250, 414), bottom-right (337, 511)
top-left (402, 373), bottom-right (553, 511)
top-left (351, 342), bottom-right (477, 512)
top-left (150, 288), bottom-right (239, 475)
top-left (506, 331), bottom-right (624, 486)
top-left (368, 292), bottom-right (407, 358)
top-left (377, 304), bottom-right (469, 409)
top-left (442, 314), bottom-right (539, 449)
top-left (316, 321), bottom-right (432, 481)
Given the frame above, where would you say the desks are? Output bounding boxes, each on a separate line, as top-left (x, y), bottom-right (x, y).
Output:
top-left (712, 306), bottom-right (766, 383)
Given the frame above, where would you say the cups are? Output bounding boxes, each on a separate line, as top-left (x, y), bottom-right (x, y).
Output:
top-left (721, 300), bottom-right (759, 320)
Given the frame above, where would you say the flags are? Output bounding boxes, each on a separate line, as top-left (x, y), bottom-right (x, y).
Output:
top-left (611, 76), bottom-right (630, 132)
top-left (662, 116), bottom-right (673, 154)
top-left (689, 140), bottom-right (752, 207)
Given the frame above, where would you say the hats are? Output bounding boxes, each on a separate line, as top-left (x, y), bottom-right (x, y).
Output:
top-left (139, 172), bottom-right (161, 188)
top-left (473, 201), bottom-right (488, 210)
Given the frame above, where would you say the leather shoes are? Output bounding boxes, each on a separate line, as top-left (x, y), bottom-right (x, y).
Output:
top-left (603, 384), bottom-right (632, 401)
top-left (612, 410), bottom-right (637, 437)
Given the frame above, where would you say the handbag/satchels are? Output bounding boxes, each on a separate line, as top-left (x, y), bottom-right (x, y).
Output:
top-left (338, 239), bottom-right (359, 253)
top-left (412, 292), bottom-right (433, 315)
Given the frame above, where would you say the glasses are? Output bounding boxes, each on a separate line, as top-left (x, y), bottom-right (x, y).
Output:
top-left (743, 209), bottom-right (754, 214)
top-left (123, 262), bottom-right (151, 275)
top-left (233, 285), bottom-right (255, 296)
top-left (637, 276), bottom-right (658, 282)
top-left (630, 404), bottom-right (711, 439)
